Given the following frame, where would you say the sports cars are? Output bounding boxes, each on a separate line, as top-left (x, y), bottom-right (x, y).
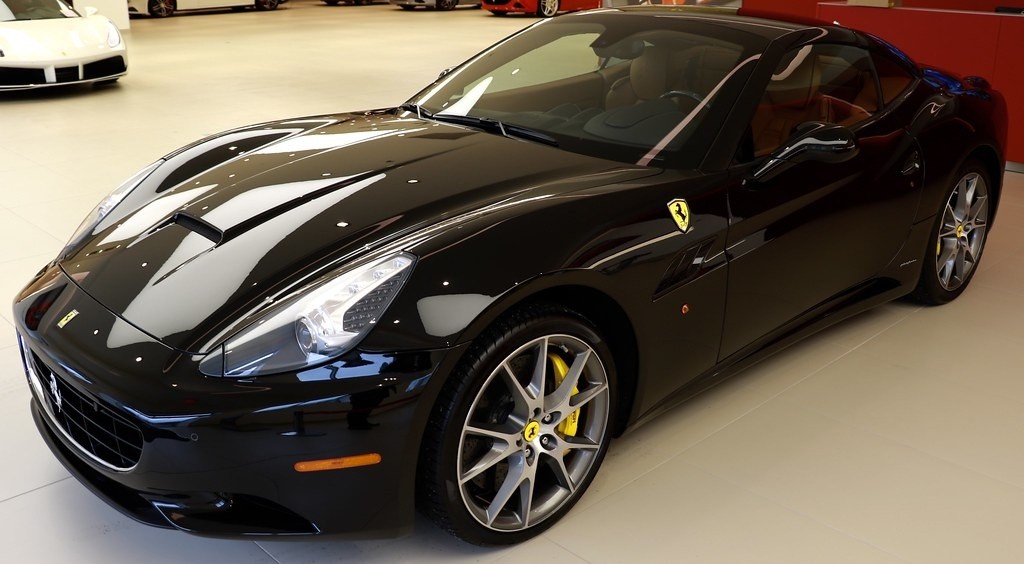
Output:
top-left (13, 3), bottom-right (1008, 550)
top-left (0, 0), bottom-right (132, 98)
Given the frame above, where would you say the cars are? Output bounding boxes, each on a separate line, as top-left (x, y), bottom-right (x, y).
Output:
top-left (127, 0), bottom-right (604, 20)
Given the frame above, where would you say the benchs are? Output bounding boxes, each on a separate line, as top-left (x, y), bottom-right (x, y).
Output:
top-left (670, 45), bottom-right (908, 127)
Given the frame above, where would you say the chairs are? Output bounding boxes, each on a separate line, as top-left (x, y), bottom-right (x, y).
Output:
top-left (751, 54), bottom-right (834, 152)
top-left (605, 46), bottom-right (690, 113)
